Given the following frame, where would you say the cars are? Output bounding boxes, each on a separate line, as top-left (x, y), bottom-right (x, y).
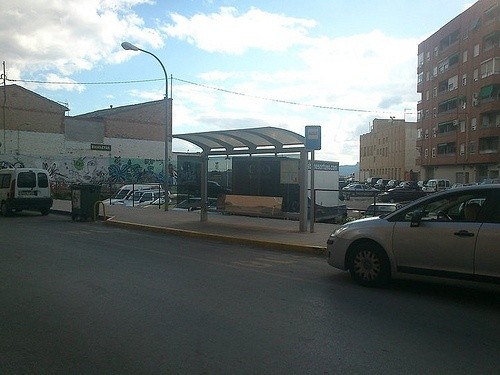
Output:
top-left (326, 183), bottom-right (500, 291)
top-left (459, 198), bottom-right (486, 214)
top-left (340, 176), bottom-right (470, 205)
top-left (360, 202), bottom-right (403, 219)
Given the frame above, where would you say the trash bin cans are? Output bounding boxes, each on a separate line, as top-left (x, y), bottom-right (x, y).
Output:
top-left (70, 182), bottom-right (102, 221)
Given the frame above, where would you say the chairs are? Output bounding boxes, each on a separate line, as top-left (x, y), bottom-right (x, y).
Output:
top-left (463, 204), bottom-right (481, 220)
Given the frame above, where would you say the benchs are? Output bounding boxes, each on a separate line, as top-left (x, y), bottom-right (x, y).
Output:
top-left (214, 193), bottom-right (285, 214)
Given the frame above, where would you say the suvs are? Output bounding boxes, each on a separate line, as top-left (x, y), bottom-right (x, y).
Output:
top-left (100, 181), bottom-right (230, 212)
top-left (0, 167), bottom-right (54, 215)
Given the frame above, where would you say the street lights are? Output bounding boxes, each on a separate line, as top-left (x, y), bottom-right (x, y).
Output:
top-left (121, 42), bottom-right (171, 211)
